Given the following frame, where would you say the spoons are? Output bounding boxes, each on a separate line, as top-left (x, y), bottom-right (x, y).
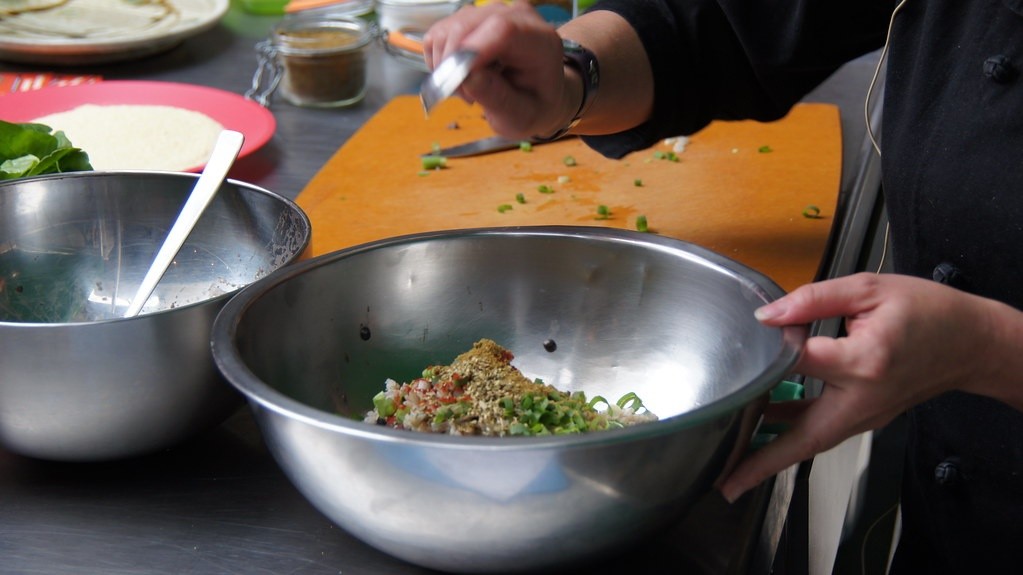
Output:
top-left (419, 50), bottom-right (475, 120)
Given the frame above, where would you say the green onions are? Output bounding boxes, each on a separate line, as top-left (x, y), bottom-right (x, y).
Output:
top-left (422, 119), bottom-right (821, 234)
top-left (372, 362), bottom-right (644, 437)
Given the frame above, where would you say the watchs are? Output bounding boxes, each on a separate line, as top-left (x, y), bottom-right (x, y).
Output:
top-left (532, 39), bottom-right (600, 142)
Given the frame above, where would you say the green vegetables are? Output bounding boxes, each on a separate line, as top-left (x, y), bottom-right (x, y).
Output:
top-left (0, 119), bottom-right (95, 181)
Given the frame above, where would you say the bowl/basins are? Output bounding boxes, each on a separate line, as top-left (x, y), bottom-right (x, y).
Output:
top-left (1, 170), bottom-right (311, 460)
top-left (208, 227), bottom-right (809, 574)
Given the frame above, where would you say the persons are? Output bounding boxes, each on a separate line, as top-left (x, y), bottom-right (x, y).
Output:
top-left (422, 0), bottom-right (1023, 575)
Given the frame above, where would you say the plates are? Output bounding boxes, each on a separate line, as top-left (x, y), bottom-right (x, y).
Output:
top-left (0, 79), bottom-right (277, 173)
top-left (0, 1), bottom-right (230, 64)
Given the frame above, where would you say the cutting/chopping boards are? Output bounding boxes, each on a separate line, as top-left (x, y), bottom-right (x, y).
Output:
top-left (291, 95), bottom-right (841, 295)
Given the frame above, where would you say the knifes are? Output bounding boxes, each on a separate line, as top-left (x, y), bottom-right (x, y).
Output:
top-left (419, 136), bottom-right (580, 158)
